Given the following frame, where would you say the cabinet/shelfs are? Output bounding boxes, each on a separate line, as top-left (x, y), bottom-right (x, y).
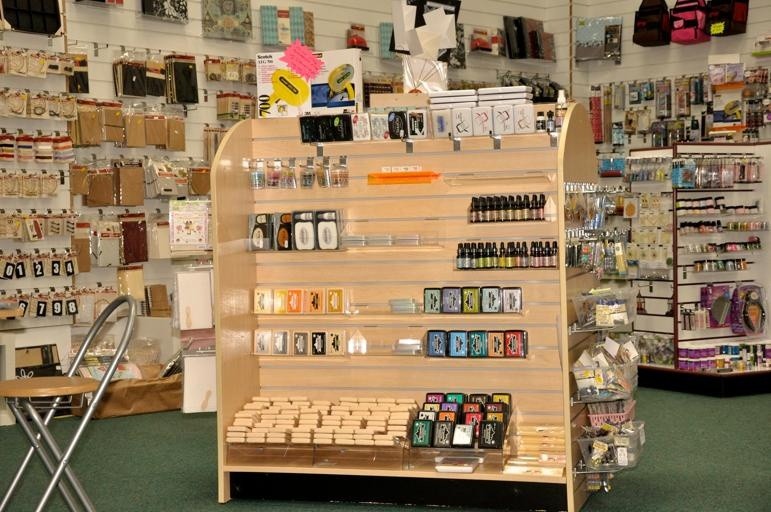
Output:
top-left (203, 100), bottom-right (624, 512)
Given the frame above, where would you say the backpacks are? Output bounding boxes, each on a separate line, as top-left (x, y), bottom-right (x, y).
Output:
top-left (704, 0), bottom-right (749, 37)
top-left (669, 0), bottom-right (711, 44)
top-left (633, 0), bottom-right (670, 45)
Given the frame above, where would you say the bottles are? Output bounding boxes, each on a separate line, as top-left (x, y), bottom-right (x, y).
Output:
top-left (536, 89), bottom-right (567, 133)
top-left (469, 194), bottom-right (546, 222)
top-left (454, 241), bottom-right (558, 269)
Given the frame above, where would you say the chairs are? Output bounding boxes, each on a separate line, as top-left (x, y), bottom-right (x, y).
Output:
top-left (1, 295), bottom-right (137, 512)
